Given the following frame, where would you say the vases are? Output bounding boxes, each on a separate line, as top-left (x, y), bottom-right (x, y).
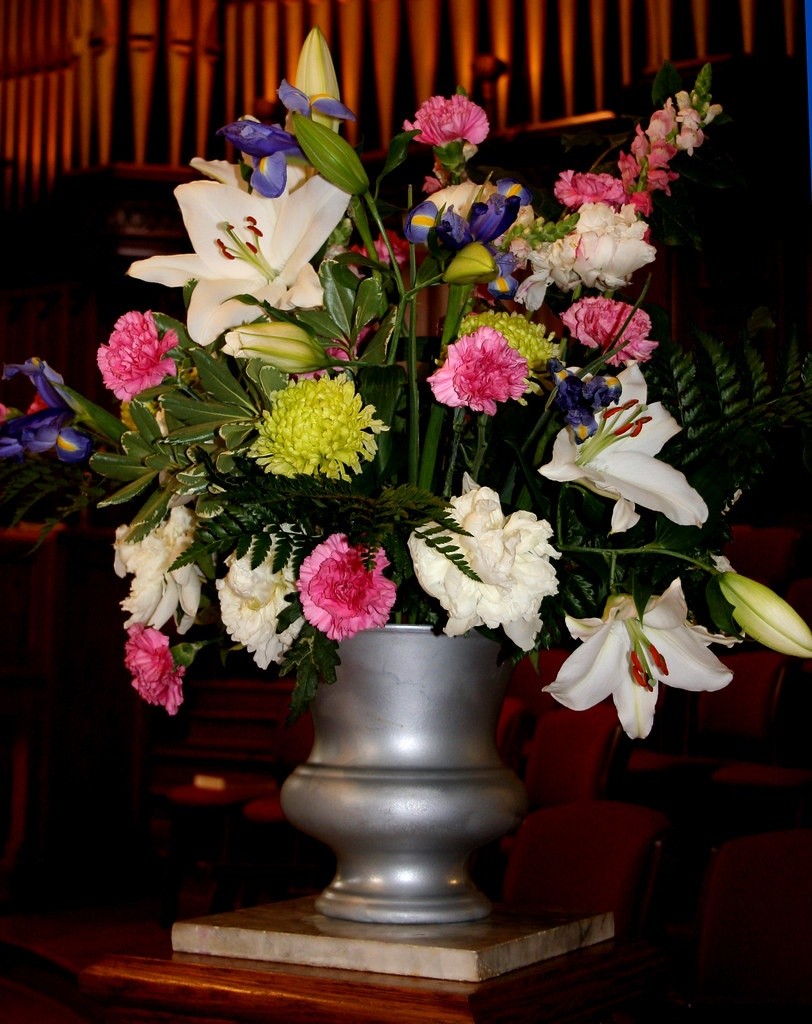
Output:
top-left (279, 620), bottom-right (521, 920)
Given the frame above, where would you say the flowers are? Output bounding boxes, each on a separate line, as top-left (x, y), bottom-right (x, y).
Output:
top-left (0, 26), bottom-right (812, 741)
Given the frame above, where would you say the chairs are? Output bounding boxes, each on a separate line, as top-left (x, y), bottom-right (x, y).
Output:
top-left (144, 500), bottom-right (812, 1024)
